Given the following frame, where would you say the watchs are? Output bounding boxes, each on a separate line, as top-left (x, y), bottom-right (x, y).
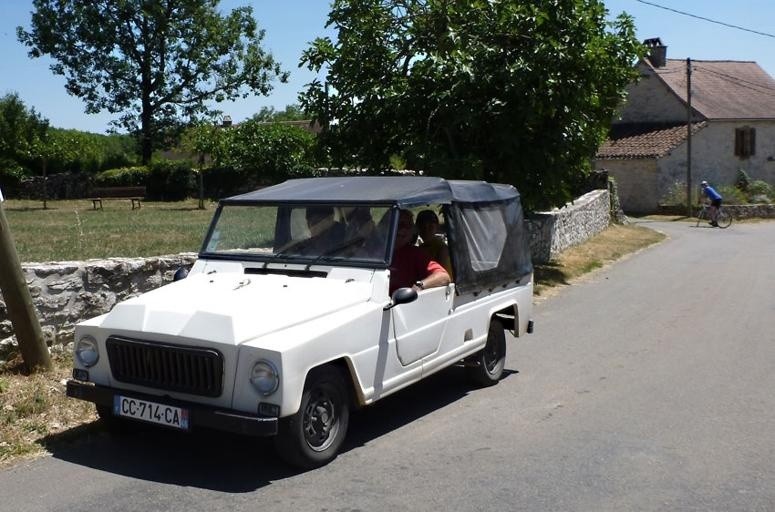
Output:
top-left (415, 280), bottom-right (425, 291)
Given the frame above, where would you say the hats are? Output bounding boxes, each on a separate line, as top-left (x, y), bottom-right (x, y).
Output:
top-left (415, 210), bottom-right (439, 224)
top-left (699, 181), bottom-right (707, 185)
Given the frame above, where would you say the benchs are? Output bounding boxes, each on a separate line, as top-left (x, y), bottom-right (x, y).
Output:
top-left (86, 186), bottom-right (146, 210)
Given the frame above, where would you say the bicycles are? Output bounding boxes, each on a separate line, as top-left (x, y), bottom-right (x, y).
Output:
top-left (697, 201), bottom-right (732, 228)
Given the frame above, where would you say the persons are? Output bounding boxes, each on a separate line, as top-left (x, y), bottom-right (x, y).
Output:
top-left (342, 208), bottom-right (375, 232)
top-left (416, 210), bottom-right (454, 283)
top-left (371, 208), bottom-right (451, 296)
top-left (299, 206), bottom-right (345, 255)
top-left (700, 181), bottom-right (722, 226)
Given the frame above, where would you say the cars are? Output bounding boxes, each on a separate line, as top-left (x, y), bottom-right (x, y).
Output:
top-left (66, 176), bottom-right (534, 468)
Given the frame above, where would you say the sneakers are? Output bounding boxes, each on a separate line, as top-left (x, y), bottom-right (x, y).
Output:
top-left (709, 221), bottom-right (719, 228)
